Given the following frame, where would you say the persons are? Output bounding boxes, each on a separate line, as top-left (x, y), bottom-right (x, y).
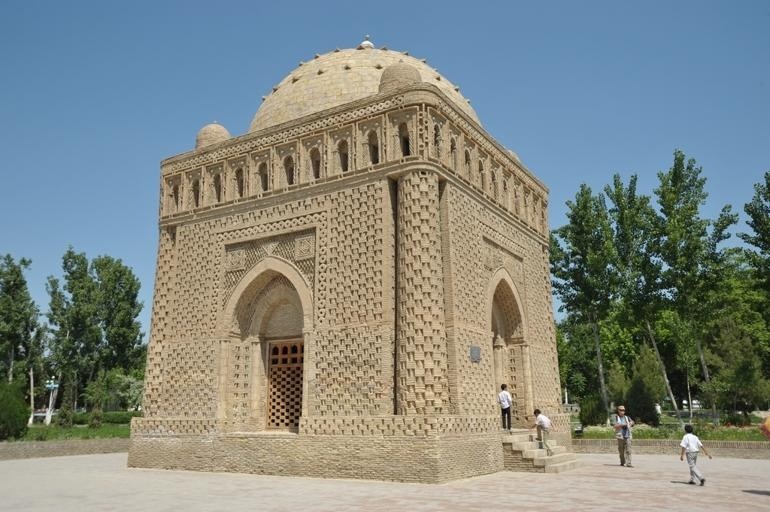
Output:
top-left (496, 383), bottom-right (512, 429)
top-left (531, 409), bottom-right (558, 456)
top-left (679, 423), bottom-right (713, 486)
top-left (612, 406), bottom-right (635, 467)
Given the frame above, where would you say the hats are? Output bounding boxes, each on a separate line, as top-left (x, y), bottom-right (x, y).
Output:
top-left (618, 406), bottom-right (626, 410)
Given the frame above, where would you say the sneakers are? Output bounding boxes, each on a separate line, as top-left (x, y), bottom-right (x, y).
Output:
top-left (536, 438), bottom-right (542, 442)
top-left (700, 479), bottom-right (704, 486)
top-left (621, 463), bottom-right (633, 467)
top-left (689, 481), bottom-right (696, 485)
